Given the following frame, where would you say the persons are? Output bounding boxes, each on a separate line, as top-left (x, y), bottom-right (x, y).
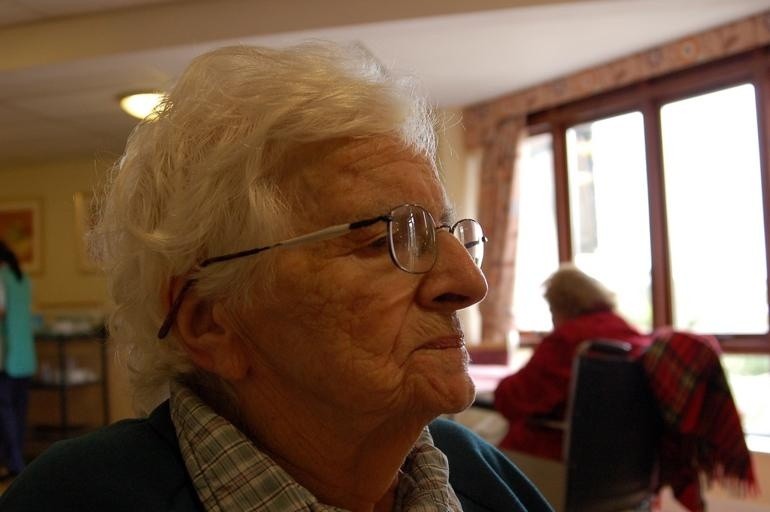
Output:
top-left (1, 38), bottom-right (560, 512)
top-left (0, 237), bottom-right (39, 482)
top-left (493, 267), bottom-right (643, 512)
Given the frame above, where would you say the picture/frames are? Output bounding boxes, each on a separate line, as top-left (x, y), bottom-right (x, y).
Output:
top-left (72, 191), bottom-right (103, 270)
top-left (0, 197), bottom-right (41, 278)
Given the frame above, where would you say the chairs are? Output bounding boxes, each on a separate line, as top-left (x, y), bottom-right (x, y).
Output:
top-left (475, 327), bottom-right (755, 512)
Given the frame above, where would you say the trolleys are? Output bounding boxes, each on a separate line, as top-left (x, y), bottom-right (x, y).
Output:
top-left (33, 316), bottom-right (112, 439)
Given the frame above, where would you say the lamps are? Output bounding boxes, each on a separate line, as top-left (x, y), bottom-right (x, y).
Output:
top-left (120, 93), bottom-right (169, 121)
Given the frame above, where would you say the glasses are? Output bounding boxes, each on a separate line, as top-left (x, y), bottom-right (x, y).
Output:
top-left (157, 204), bottom-right (488, 338)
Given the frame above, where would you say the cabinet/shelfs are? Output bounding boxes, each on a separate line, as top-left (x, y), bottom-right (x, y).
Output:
top-left (29, 322), bottom-right (108, 450)
